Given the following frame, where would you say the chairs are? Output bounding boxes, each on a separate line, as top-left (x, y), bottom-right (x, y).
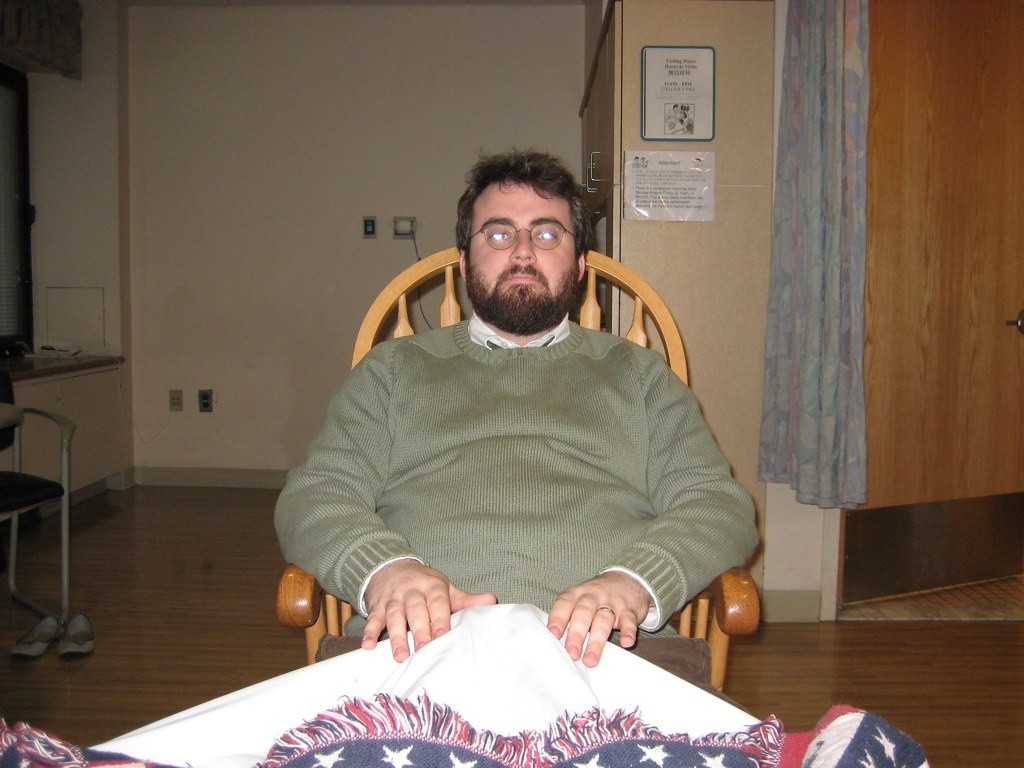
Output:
top-left (0, 364), bottom-right (77, 623)
top-left (276, 244), bottom-right (761, 690)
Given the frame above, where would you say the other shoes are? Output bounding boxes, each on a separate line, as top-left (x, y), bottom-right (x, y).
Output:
top-left (8, 615), bottom-right (64, 659)
top-left (58, 614), bottom-right (95, 660)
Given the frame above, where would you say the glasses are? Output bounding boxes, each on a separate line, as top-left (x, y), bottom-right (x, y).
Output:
top-left (469, 219), bottom-right (576, 250)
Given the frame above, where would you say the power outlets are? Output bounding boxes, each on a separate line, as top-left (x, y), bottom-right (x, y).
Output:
top-left (393, 216), bottom-right (417, 240)
top-left (169, 390), bottom-right (183, 411)
top-left (197, 389), bottom-right (214, 413)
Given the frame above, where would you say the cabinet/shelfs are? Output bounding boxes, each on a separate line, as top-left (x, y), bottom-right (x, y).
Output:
top-left (580, 0), bottom-right (776, 592)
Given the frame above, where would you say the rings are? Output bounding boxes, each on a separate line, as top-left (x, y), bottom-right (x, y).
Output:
top-left (596, 606), bottom-right (617, 622)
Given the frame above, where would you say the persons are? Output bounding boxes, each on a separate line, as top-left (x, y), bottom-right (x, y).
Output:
top-left (274, 145), bottom-right (757, 666)
